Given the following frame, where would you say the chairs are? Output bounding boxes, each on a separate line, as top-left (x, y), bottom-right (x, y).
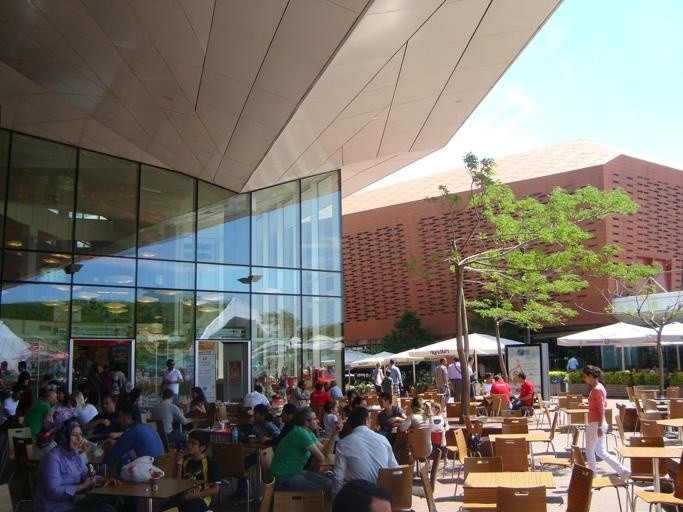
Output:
top-left (0, 384), bottom-right (683, 512)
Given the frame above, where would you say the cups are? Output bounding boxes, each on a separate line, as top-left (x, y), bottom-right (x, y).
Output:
top-left (149, 478), bottom-right (162, 495)
top-left (247, 434), bottom-right (256, 445)
top-left (230, 424), bottom-right (238, 443)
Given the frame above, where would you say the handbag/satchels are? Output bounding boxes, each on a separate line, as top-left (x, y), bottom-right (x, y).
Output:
top-left (468, 432), bottom-right (481, 452)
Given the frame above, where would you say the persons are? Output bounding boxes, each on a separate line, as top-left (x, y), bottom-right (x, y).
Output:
top-left (242, 357), bottom-right (450, 512)
top-left (435, 358), bottom-right (534, 414)
top-left (566, 358), bottom-right (578, 370)
top-left (582, 365), bottom-right (632, 483)
top-left (1, 356), bottom-right (222, 512)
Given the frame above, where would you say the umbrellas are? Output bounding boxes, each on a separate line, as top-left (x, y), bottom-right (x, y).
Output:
top-left (252, 335), bottom-right (344, 376)
top-left (556, 322), bottom-right (654, 371)
top-left (646, 322), bottom-right (683, 371)
top-left (390, 348), bottom-right (425, 383)
top-left (351, 351), bottom-right (398, 375)
top-left (408, 332), bottom-right (526, 382)
top-left (325, 350), bottom-right (372, 384)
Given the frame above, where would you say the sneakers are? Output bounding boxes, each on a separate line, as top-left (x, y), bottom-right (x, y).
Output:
top-left (621, 469), bottom-right (632, 481)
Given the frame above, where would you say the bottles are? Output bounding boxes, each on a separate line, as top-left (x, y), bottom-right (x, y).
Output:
top-left (226, 428), bottom-right (231, 442)
